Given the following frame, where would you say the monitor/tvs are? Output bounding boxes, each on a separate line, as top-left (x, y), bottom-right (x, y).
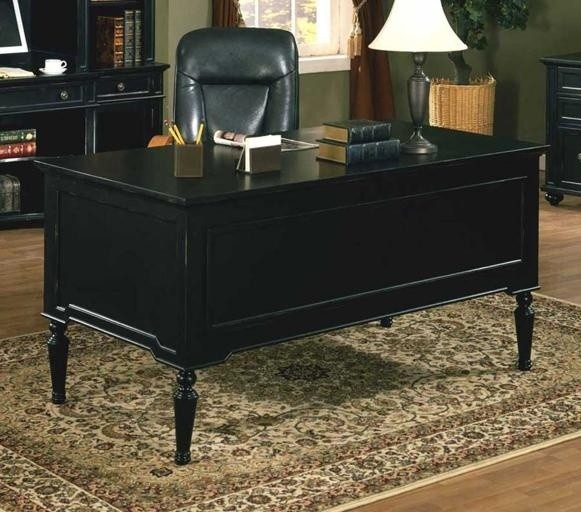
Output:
top-left (0, 0), bottom-right (29, 54)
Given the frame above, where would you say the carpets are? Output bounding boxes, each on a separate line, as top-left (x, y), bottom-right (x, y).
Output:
top-left (1, 289), bottom-right (580, 512)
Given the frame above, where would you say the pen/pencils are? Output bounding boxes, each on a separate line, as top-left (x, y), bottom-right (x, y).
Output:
top-left (165, 119), bottom-right (206, 145)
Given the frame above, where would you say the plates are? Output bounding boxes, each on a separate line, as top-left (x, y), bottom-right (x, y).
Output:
top-left (39, 67), bottom-right (67, 75)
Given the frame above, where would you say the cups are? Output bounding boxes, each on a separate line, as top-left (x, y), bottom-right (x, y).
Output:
top-left (45, 59), bottom-right (66, 71)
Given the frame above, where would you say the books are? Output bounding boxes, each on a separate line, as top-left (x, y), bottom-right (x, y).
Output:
top-left (316, 136), bottom-right (403, 165)
top-left (134, 10), bottom-right (143, 62)
top-left (214, 129), bottom-right (319, 153)
top-left (1, 128), bottom-right (37, 145)
top-left (122, 10), bottom-right (135, 67)
top-left (0, 141), bottom-right (37, 159)
top-left (0, 65), bottom-right (36, 79)
top-left (322, 117), bottom-right (393, 144)
top-left (93, 15), bottom-right (125, 67)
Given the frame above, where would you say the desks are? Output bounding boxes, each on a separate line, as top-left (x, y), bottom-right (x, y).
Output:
top-left (32, 115), bottom-right (549, 466)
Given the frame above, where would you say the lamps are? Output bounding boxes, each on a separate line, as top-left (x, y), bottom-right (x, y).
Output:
top-left (365, 0), bottom-right (472, 158)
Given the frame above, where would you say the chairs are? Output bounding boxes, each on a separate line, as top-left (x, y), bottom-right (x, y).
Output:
top-left (171, 25), bottom-right (298, 145)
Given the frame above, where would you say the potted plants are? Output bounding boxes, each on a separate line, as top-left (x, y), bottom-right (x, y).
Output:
top-left (427, 0), bottom-right (528, 136)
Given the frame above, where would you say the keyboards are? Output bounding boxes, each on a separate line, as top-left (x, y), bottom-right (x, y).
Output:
top-left (0, 67), bottom-right (34, 78)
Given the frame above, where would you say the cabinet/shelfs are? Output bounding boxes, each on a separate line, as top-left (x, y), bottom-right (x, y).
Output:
top-left (536, 53), bottom-right (581, 207)
top-left (0, 0), bottom-right (171, 230)
